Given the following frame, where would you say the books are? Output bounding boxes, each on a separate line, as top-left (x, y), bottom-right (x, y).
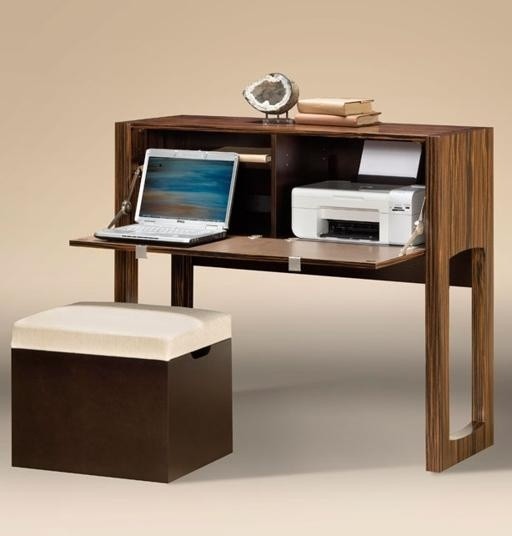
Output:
top-left (294, 111), bottom-right (383, 127)
top-left (215, 145), bottom-right (273, 164)
top-left (296, 98), bottom-right (376, 117)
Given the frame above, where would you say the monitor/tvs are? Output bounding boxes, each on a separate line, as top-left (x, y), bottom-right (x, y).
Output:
top-left (93, 148), bottom-right (240, 247)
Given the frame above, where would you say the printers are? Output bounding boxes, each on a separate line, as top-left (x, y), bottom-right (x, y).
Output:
top-left (290, 181), bottom-right (426, 245)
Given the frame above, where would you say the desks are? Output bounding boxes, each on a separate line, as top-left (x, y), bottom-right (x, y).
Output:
top-left (68, 113), bottom-right (496, 473)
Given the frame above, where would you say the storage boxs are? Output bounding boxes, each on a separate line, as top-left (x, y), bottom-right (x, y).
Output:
top-left (11, 300), bottom-right (232, 484)
